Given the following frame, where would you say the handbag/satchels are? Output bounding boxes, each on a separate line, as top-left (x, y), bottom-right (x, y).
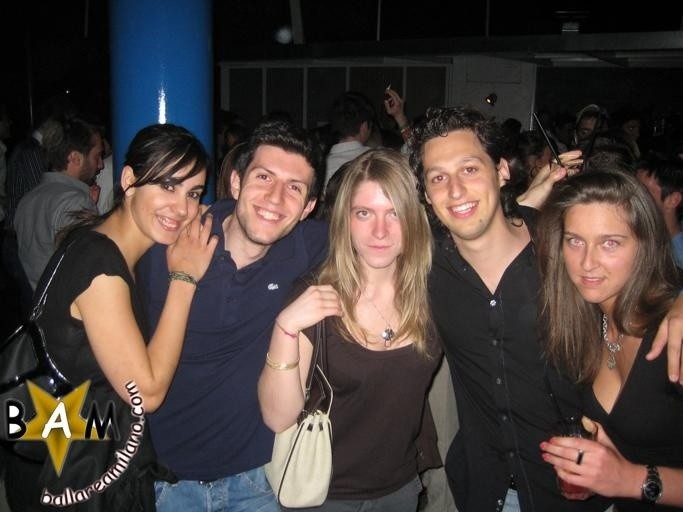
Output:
top-left (1, 319), bottom-right (157, 511)
top-left (264, 410), bottom-right (333, 509)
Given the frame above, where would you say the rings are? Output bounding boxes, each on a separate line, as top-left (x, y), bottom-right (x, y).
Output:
top-left (574, 449), bottom-right (584, 467)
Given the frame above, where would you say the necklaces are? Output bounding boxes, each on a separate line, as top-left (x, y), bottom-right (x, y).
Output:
top-left (355, 279), bottom-right (408, 347)
top-left (600, 313), bottom-right (625, 372)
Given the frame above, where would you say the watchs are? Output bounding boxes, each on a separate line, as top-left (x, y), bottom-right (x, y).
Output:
top-left (639, 463), bottom-right (663, 507)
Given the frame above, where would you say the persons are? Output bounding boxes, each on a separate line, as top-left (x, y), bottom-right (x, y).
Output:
top-left (535, 165), bottom-right (681, 512)
top-left (404, 103), bottom-right (680, 511)
top-left (21, 121), bottom-right (223, 512)
top-left (130, 117), bottom-right (584, 512)
top-left (1, 82), bottom-right (683, 316)
top-left (255, 145), bottom-right (446, 512)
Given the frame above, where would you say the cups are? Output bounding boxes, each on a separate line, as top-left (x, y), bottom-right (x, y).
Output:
top-left (551, 415), bottom-right (599, 502)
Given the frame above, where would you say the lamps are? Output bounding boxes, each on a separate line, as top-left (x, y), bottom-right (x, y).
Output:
top-left (486, 93), bottom-right (497, 106)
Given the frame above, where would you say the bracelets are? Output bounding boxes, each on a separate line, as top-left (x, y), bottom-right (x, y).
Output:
top-left (262, 350), bottom-right (301, 375)
top-left (166, 270), bottom-right (200, 288)
top-left (271, 316), bottom-right (301, 341)
